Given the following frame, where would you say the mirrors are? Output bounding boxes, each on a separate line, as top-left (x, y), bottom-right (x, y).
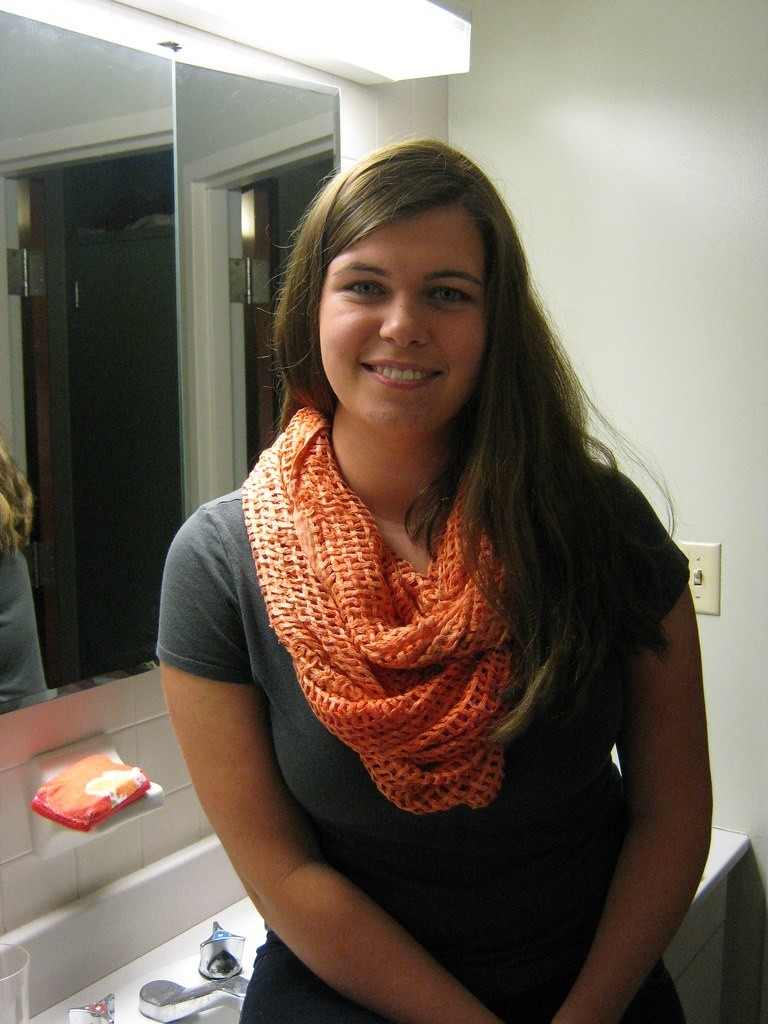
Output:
top-left (0, 8), bottom-right (340, 715)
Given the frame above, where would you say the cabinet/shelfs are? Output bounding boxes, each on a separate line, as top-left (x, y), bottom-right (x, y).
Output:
top-left (0, 829), bottom-right (750, 1024)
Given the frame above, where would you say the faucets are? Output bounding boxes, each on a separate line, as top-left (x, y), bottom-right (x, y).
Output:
top-left (139, 975), bottom-right (262, 1024)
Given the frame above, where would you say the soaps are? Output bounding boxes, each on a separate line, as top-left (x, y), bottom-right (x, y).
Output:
top-left (31, 752), bottom-right (152, 833)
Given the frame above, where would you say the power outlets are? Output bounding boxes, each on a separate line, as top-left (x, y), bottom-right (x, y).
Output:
top-left (674, 539), bottom-right (724, 616)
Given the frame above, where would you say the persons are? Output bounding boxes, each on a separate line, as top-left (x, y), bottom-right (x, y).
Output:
top-left (0, 442), bottom-right (52, 703)
top-left (146, 137), bottom-right (719, 1024)
